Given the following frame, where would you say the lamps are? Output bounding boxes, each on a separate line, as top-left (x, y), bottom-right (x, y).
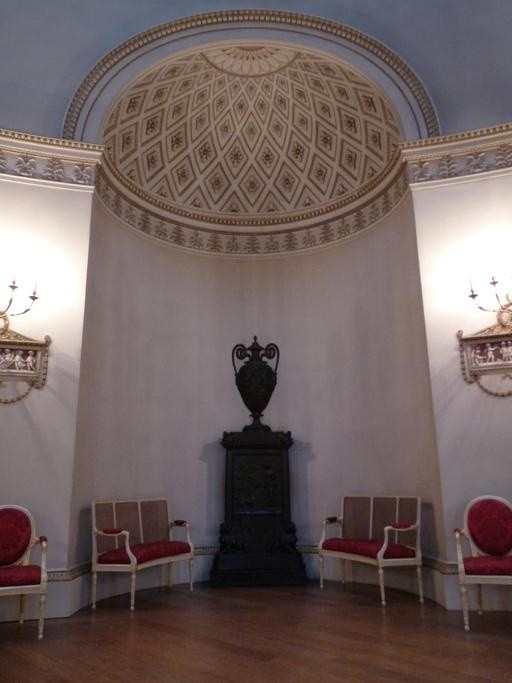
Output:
top-left (457, 274), bottom-right (511, 399)
top-left (1, 277), bottom-right (52, 405)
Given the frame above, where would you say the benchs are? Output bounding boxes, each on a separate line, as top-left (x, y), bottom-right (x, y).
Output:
top-left (88, 498), bottom-right (195, 610)
top-left (317, 493), bottom-right (423, 605)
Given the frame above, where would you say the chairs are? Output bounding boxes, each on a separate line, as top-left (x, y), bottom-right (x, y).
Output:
top-left (1, 504), bottom-right (50, 642)
top-left (454, 496), bottom-right (511, 629)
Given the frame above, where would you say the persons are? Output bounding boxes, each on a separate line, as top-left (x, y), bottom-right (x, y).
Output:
top-left (473, 340), bottom-right (511, 366)
top-left (0, 347), bottom-right (38, 371)
